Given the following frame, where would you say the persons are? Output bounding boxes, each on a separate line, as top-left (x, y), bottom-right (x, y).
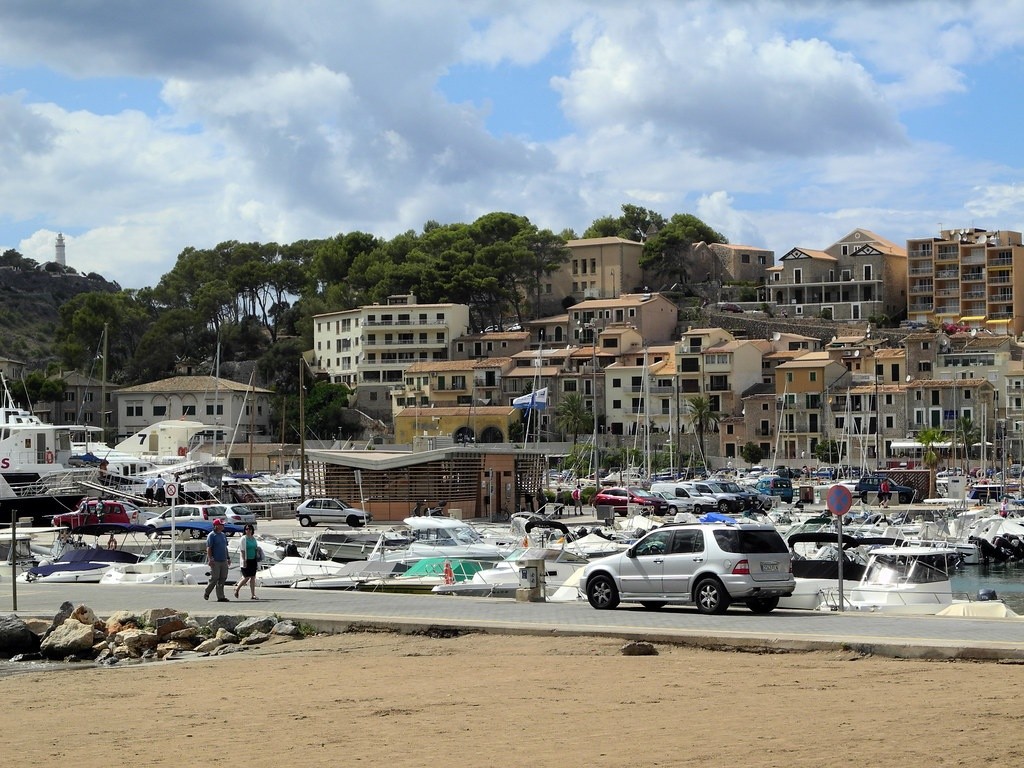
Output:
top-left (555, 487), bottom-right (564, 519)
top-left (572, 484), bottom-right (584, 516)
top-left (144, 476), bottom-right (156, 507)
top-left (203, 519), bottom-right (232, 602)
top-left (877, 479), bottom-right (892, 509)
top-left (1000, 497), bottom-right (1008, 517)
top-left (234, 523), bottom-right (260, 600)
top-left (149, 474), bottom-right (166, 507)
top-left (95, 496), bottom-right (105, 524)
top-left (78, 497), bottom-right (91, 526)
top-left (536, 487), bottom-right (546, 516)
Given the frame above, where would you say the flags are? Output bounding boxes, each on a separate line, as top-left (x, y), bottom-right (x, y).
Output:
top-left (512, 387), bottom-right (549, 409)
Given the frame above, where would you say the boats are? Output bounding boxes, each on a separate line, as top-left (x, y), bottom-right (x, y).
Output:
top-left (102, 520), bottom-right (296, 585)
top-left (288, 537), bottom-right (520, 592)
top-left (512, 332), bottom-right (1024, 603)
top-left (429, 546), bottom-right (592, 600)
top-left (0, 322), bottom-right (328, 528)
top-left (229, 514), bottom-right (425, 587)
top-left (15, 522), bottom-right (155, 584)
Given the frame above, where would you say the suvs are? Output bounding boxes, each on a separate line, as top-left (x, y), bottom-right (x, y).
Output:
top-left (295, 497), bottom-right (374, 528)
top-left (211, 504), bottom-right (258, 537)
top-left (593, 486), bottom-right (667, 518)
top-left (855, 477), bottom-right (915, 504)
top-left (578, 520), bottom-right (796, 616)
top-left (144, 504), bottom-right (229, 539)
top-left (680, 481), bottom-right (743, 514)
top-left (727, 481), bottom-right (773, 512)
top-left (651, 483), bottom-right (719, 514)
top-left (51, 500), bottom-right (160, 535)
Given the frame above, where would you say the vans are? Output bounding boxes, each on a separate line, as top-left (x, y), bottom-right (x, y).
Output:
top-left (755, 477), bottom-right (793, 505)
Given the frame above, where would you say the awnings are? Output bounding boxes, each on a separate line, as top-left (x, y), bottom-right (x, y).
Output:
top-left (890, 440), bottom-right (964, 449)
top-left (907, 310), bottom-right (1011, 324)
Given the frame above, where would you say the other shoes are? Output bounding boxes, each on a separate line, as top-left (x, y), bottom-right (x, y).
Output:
top-left (218, 596), bottom-right (229, 602)
top-left (234, 588), bottom-right (238, 598)
top-left (204, 589), bottom-right (209, 601)
top-left (251, 595), bottom-right (259, 600)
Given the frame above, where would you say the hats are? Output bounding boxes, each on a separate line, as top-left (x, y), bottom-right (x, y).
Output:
top-left (213, 519), bottom-right (225, 525)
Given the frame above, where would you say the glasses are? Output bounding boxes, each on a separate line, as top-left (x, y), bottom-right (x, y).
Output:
top-left (246, 528), bottom-right (252, 530)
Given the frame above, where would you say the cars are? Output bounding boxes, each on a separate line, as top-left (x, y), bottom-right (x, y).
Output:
top-left (646, 490), bottom-right (694, 516)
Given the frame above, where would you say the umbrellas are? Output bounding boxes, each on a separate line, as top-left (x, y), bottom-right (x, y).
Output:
top-left (972, 441), bottom-right (993, 446)
